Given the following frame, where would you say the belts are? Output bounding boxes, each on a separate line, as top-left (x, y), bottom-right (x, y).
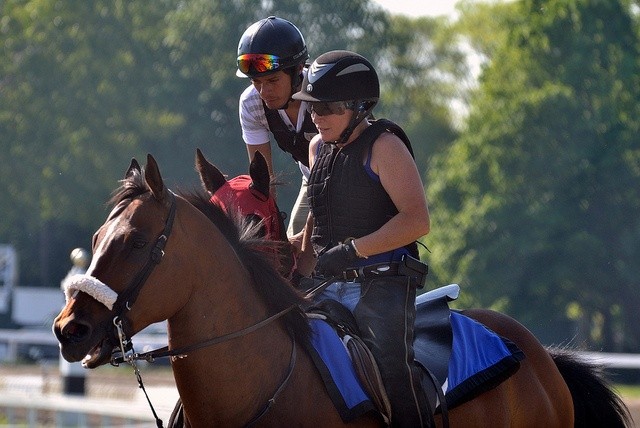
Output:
top-left (340, 264), bottom-right (400, 279)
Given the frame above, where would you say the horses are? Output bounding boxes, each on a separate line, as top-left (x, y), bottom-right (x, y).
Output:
top-left (51, 153), bottom-right (639, 428)
top-left (195, 147), bottom-right (296, 279)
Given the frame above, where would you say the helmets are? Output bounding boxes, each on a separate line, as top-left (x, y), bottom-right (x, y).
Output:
top-left (292, 51), bottom-right (379, 103)
top-left (236, 16), bottom-right (310, 79)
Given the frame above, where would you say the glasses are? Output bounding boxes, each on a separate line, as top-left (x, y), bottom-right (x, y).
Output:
top-left (236, 47), bottom-right (307, 74)
top-left (305, 101), bottom-right (354, 115)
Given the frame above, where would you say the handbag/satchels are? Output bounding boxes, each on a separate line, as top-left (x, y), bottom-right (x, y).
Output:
top-left (398, 253), bottom-right (428, 289)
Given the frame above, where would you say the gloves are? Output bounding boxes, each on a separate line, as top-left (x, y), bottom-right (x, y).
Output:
top-left (315, 237), bottom-right (361, 277)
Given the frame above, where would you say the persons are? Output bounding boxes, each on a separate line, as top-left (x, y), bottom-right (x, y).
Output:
top-left (236, 16), bottom-right (321, 246)
top-left (290, 50), bottom-right (436, 427)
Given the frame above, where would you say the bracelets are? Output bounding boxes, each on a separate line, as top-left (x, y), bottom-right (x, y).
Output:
top-left (350, 239), bottom-right (369, 261)
